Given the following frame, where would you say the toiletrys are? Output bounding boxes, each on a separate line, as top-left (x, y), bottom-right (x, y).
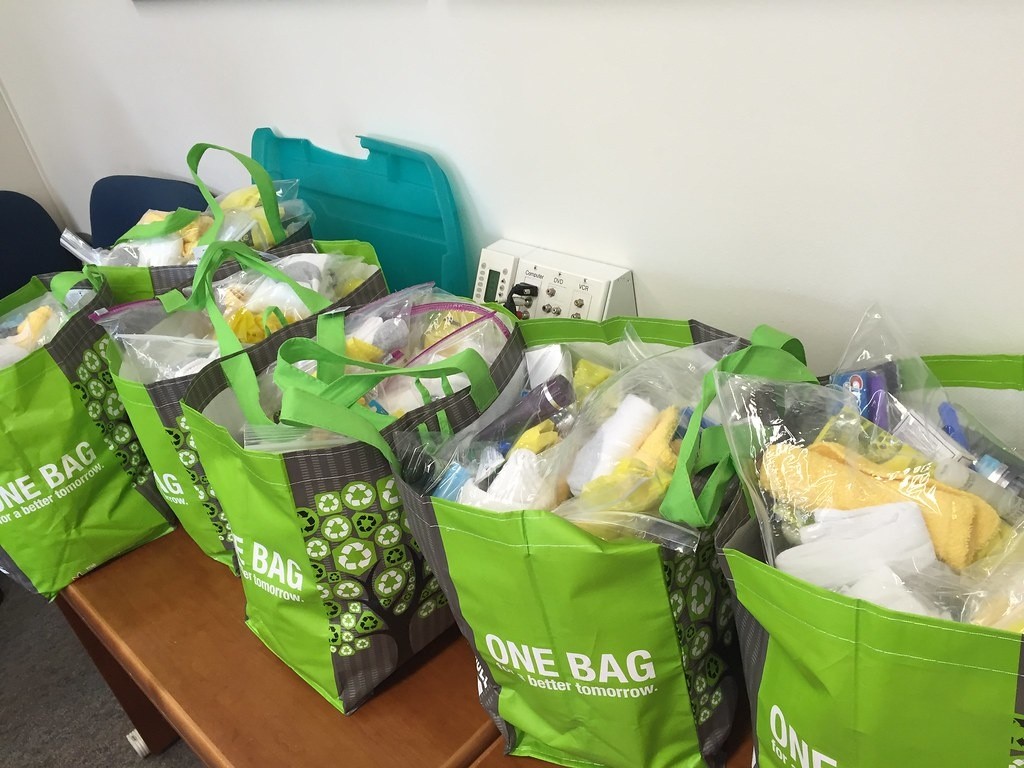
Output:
top-left (402, 343), bottom-right (662, 503)
top-left (733, 354), bottom-right (1022, 518)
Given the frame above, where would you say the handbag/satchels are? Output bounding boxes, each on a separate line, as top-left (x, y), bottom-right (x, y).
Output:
top-left (107, 239), bottom-right (390, 578)
top-left (82, 144), bottom-right (313, 303)
top-left (659, 345), bottom-right (1024, 768)
top-left (179, 287), bottom-right (521, 717)
top-left (278, 316), bottom-right (807, 768)
top-left (0, 263), bottom-right (179, 605)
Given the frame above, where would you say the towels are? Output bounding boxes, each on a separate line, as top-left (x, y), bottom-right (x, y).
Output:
top-left (456, 391), bottom-right (688, 544)
top-left (14, 303), bottom-right (58, 351)
top-left (753, 436), bottom-right (1023, 635)
top-left (134, 181), bottom-right (283, 266)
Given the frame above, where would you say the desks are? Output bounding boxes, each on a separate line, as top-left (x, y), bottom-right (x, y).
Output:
top-left (54, 518), bottom-right (757, 768)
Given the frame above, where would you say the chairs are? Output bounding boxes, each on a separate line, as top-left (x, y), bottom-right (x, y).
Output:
top-left (0, 175), bottom-right (84, 303)
top-left (90, 175), bottom-right (220, 250)
